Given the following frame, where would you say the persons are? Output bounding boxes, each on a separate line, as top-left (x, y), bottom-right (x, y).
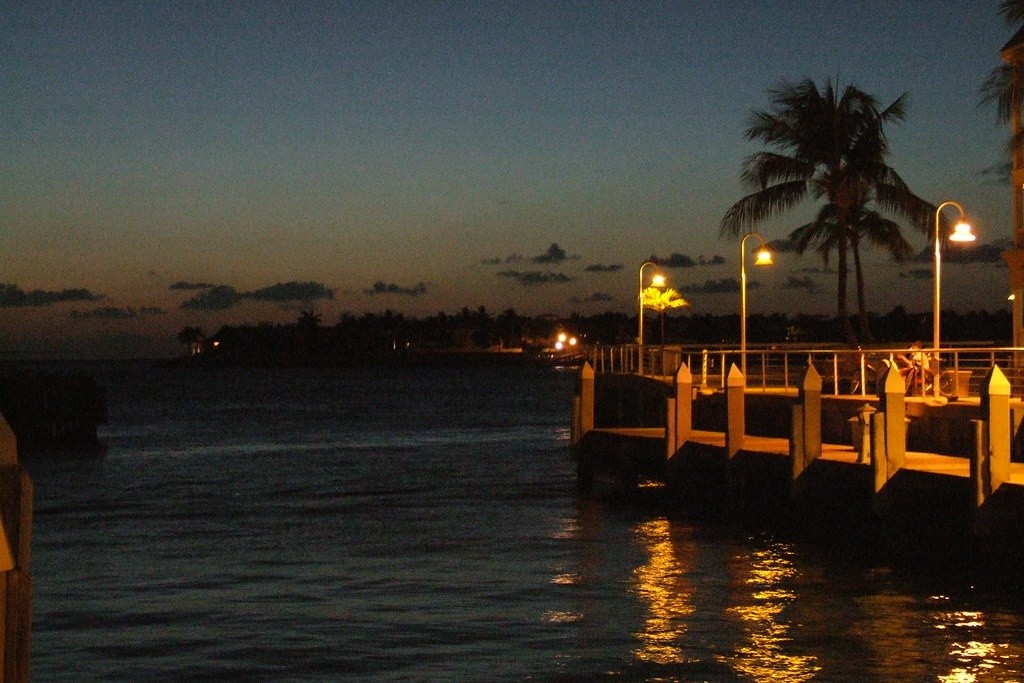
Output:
top-left (899, 341), bottom-right (931, 370)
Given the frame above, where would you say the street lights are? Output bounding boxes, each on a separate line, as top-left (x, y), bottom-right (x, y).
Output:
top-left (636, 262), bottom-right (667, 376)
top-left (741, 233), bottom-right (774, 384)
top-left (931, 200), bottom-right (976, 400)
